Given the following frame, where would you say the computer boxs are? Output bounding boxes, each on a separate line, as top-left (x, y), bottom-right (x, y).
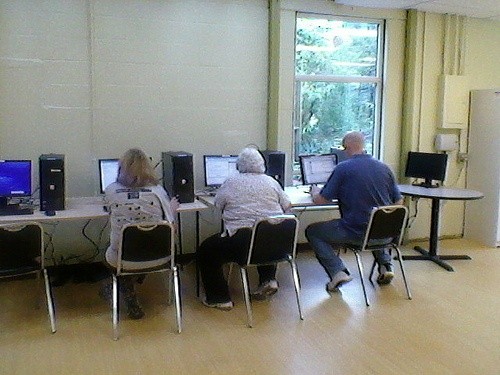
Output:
top-left (261, 150), bottom-right (285, 190)
top-left (39, 153), bottom-right (65, 210)
top-left (331, 147), bottom-right (367, 163)
top-left (162, 151), bottom-right (194, 204)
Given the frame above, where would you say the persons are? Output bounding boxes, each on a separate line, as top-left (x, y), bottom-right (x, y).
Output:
top-left (200, 147), bottom-right (293, 310)
top-left (99, 148), bottom-right (181, 320)
top-left (305, 131), bottom-right (404, 292)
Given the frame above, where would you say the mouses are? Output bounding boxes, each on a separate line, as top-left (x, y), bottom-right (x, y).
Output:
top-left (45, 210), bottom-right (55, 216)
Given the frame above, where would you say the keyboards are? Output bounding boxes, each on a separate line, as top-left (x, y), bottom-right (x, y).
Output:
top-left (0, 208), bottom-right (33, 216)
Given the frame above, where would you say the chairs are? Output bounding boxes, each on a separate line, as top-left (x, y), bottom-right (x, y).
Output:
top-left (227, 215), bottom-right (304, 328)
top-left (0, 222), bottom-right (57, 335)
top-left (334, 205), bottom-right (414, 306)
top-left (111, 221), bottom-right (183, 341)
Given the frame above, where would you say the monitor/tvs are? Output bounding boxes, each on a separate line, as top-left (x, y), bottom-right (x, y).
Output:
top-left (204, 154), bottom-right (239, 191)
top-left (405, 152), bottom-right (448, 187)
top-left (299, 154), bottom-right (338, 193)
top-left (0, 159), bottom-right (31, 208)
top-left (99, 159), bottom-right (120, 194)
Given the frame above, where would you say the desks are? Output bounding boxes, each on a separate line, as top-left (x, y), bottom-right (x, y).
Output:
top-left (0, 192), bottom-right (209, 298)
top-left (393, 185), bottom-right (484, 272)
top-left (195, 184), bottom-right (338, 301)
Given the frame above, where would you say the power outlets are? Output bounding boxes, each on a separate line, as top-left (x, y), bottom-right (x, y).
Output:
top-left (54, 256), bottom-right (81, 266)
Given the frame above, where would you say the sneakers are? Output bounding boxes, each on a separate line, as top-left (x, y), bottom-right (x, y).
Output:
top-left (327, 269), bottom-right (352, 291)
top-left (251, 279), bottom-right (278, 301)
top-left (378, 264), bottom-right (395, 284)
top-left (202, 297), bottom-right (233, 309)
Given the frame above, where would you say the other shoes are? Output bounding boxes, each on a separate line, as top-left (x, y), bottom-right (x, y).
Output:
top-left (103, 283), bottom-right (112, 306)
top-left (126, 296), bottom-right (144, 317)
